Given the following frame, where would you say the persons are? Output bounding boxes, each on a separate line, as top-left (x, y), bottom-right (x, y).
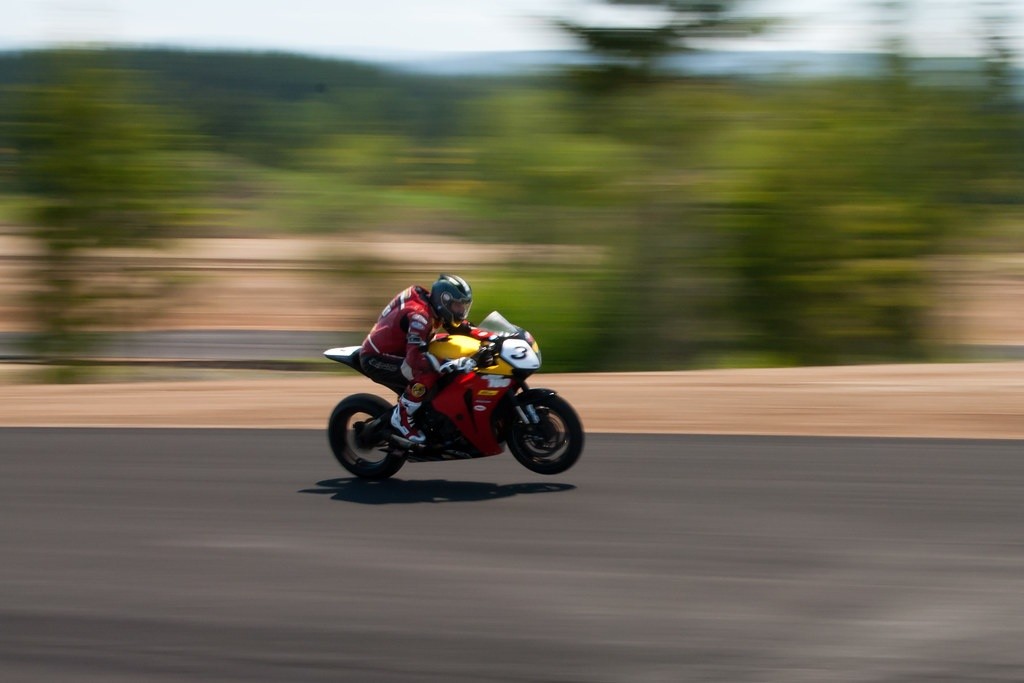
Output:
top-left (360, 274), bottom-right (518, 444)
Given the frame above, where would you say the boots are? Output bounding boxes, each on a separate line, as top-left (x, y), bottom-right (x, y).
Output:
top-left (389, 391), bottom-right (427, 444)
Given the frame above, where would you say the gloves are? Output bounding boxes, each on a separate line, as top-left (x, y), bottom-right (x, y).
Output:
top-left (439, 356), bottom-right (478, 374)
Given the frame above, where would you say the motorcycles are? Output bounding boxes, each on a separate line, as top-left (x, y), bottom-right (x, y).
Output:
top-left (322, 310), bottom-right (586, 484)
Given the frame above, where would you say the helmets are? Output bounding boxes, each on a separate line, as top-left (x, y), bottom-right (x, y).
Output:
top-left (431, 272), bottom-right (471, 327)
